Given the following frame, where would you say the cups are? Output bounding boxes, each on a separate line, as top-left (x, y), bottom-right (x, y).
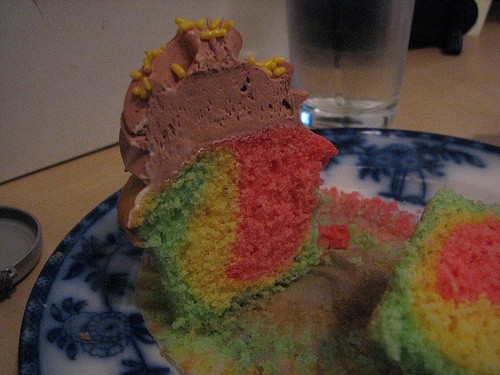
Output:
top-left (283, 1), bottom-right (414, 129)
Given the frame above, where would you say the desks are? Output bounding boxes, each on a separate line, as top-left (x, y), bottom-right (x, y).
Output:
top-left (0, 19), bottom-right (499, 374)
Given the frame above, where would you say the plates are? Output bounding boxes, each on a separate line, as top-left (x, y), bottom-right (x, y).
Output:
top-left (15, 122), bottom-right (498, 375)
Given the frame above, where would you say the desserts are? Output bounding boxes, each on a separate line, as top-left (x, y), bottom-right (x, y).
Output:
top-left (114, 14), bottom-right (337, 329)
top-left (369, 187), bottom-right (500, 374)
